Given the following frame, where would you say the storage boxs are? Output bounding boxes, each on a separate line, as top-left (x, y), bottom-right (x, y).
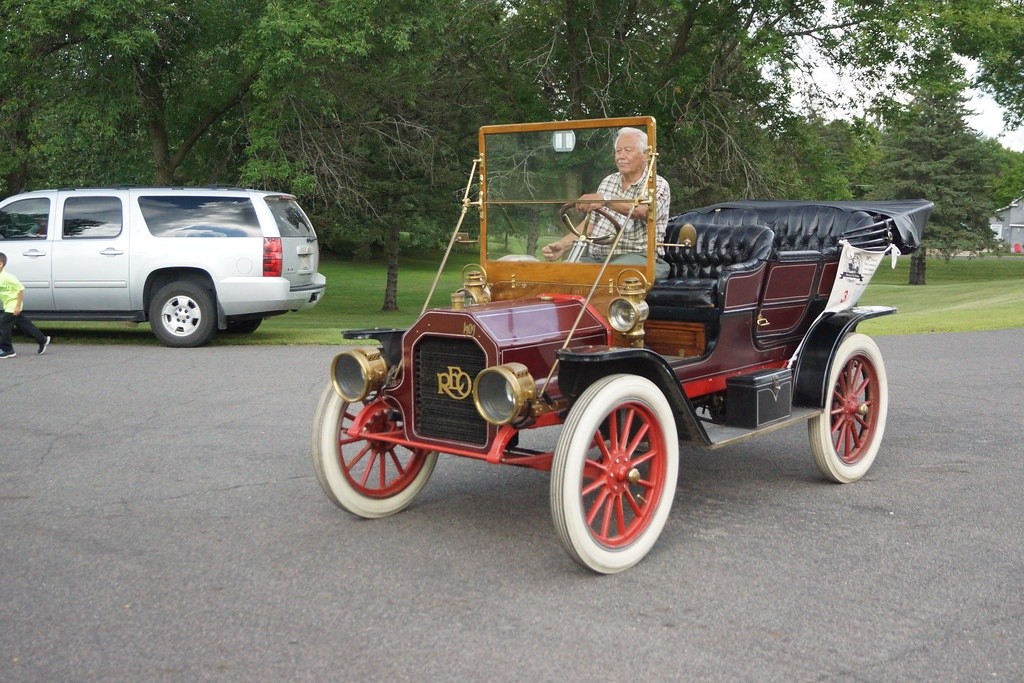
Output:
top-left (726, 368), bottom-right (792, 429)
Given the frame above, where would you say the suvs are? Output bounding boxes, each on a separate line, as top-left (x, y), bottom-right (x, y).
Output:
top-left (0, 185), bottom-right (327, 349)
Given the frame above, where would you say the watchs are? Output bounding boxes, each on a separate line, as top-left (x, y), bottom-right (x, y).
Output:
top-left (602, 193), bottom-right (611, 206)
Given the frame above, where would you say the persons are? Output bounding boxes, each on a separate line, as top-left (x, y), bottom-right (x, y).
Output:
top-left (0, 252), bottom-right (50, 360)
top-left (542, 127), bottom-right (671, 279)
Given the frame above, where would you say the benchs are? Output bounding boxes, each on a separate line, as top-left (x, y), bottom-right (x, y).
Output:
top-left (671, 204), bottom-right (876, 258)
top-left (646, 224), bottom-right (776, 321)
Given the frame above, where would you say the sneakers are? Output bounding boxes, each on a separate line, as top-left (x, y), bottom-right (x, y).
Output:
top-left (38, 336), bottom-right (51, 354)
top-left (0, 350), bottom-right (16, 359)
